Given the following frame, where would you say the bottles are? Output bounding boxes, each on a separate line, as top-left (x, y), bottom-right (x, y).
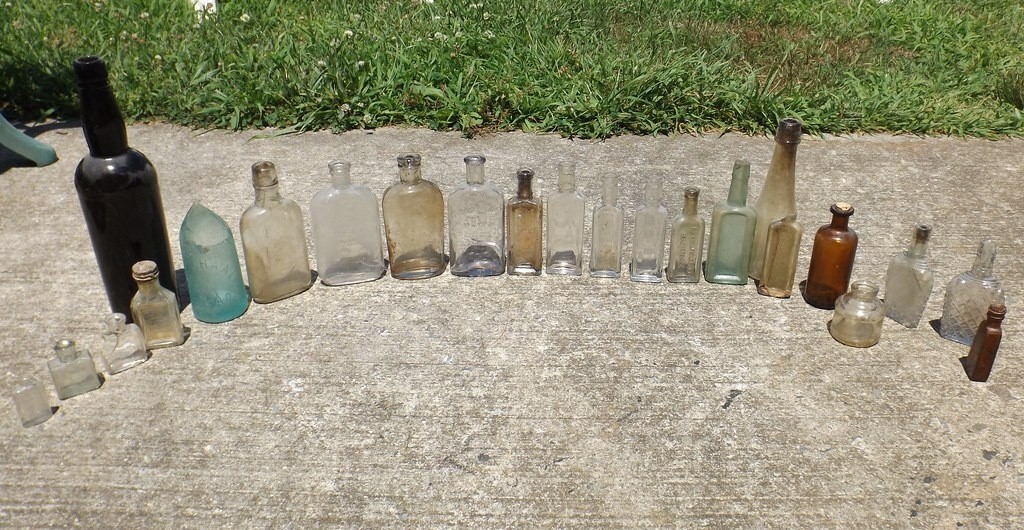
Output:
top-left (965, 305), bottom-right (1007, 383)
top-left (746, 117), bottom-right (802, 281)
top-left (667, 186), bottom-right (705, 283)
top-left (309, 159), bottom-right (384, 286)
top-left (804, 202), bottom-right (858, 309)
top-left (546, 161), bottom-right (585, 276)
top-left (705, 160), bottom-right (758, 285)
top-left (758, 210), bottom-right (802, 297)
top-left (179, 203), bottom-right (250, 324)
top-left (8, 260), bottom-right (185, 430)
top-left (240, 160), bottom-right (312, 304)
top-left (506, 167), bottom-right (543, 277)
top-left (382, 152), bottom-right (445, 280)
top-left (74, 56), bottom-right (181, 324)
top-left (939, 240), bottom-right (1005, 344)
top-left (629, 174), bottom-right (668, 283)
top-left (829, 280), bottom-right (885, 349)
top-left (587, 172), bottom-right (624, 279)
top-left (883, 223), bottom-right (934, 328)
top-left (447, 155), bottom-right (505, 276)
